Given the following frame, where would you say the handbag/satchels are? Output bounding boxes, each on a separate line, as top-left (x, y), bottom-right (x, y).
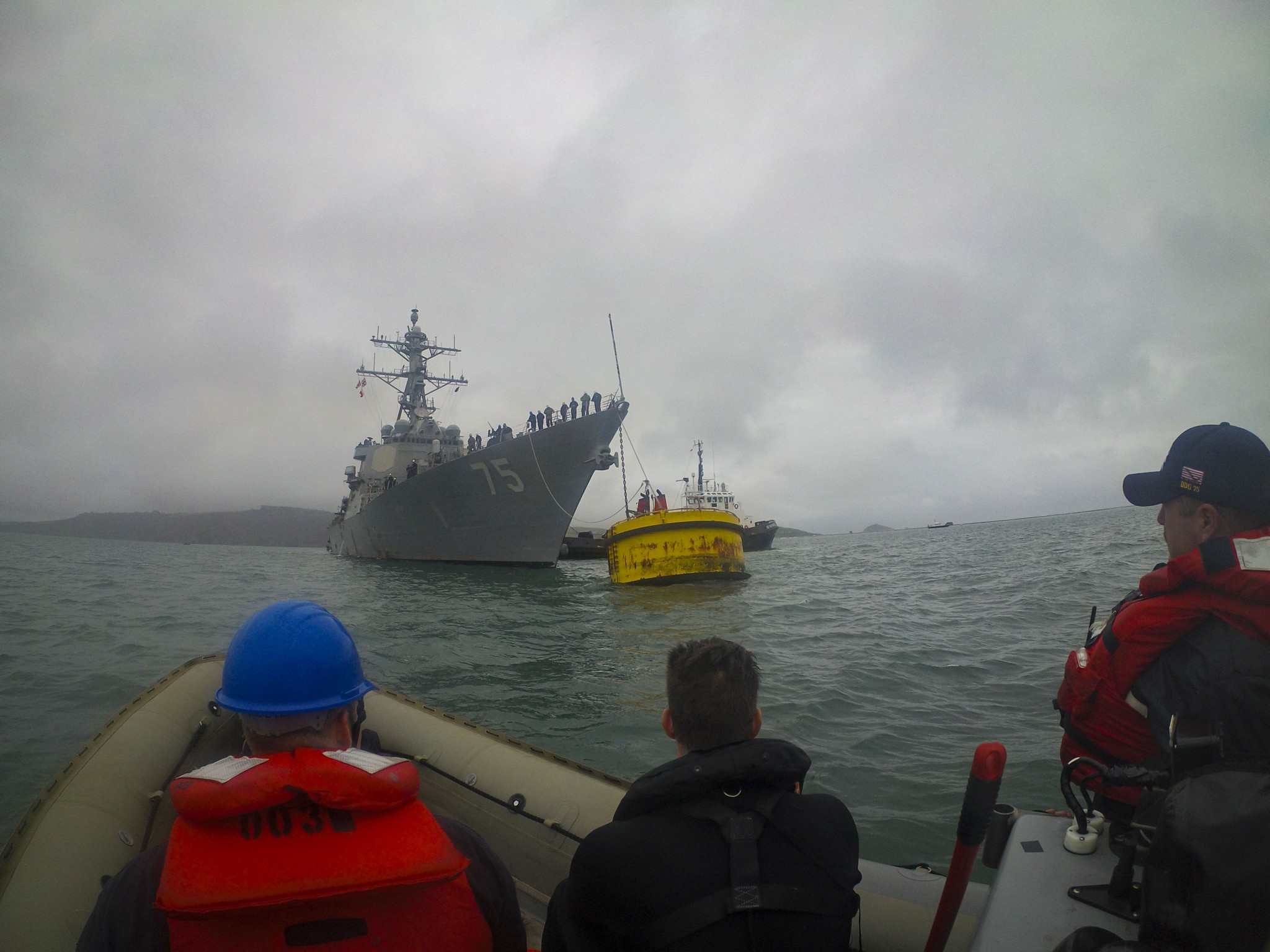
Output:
top-left (1054, 761), bottom-right (1270, 952)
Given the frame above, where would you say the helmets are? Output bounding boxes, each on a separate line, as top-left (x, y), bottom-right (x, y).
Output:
top-left (389, 473), bottom-right (391, 475)
top-left (215, 603), bottom-right (379, 715)
top-left (412, 460), bottom-right (415, 462)
top-left (394, 478), bottom-right (396, 479)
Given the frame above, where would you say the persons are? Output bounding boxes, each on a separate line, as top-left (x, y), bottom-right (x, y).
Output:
top-left (537, 410), bottom-right (545, 430)
top-left (580, 392), bottom-right (591, 416)
top-left (527, 411), bottom-right (536, 432)
top-left (384, 473), bottom-right (398, 491)
top-left (569, 397), bottom-right (578, 420)
top-left (560, 402), bottom-right (569, 423)
top-left (1062, 421), bottom-right (1269, 801)
top-left (591, 391), bottom-right (602, 412)
top-left (495, 423), bottom-right (513, 443)
top-left (468, 433), bottom-right (481, 453)
top-left (539, 635), bottom-right (863, 952)
top-left (71, 600), bottom-right (529, 951)
top-left (405, 459), bottom-right (418, 479)
top-left (544, 405), bottom-right (555, 427)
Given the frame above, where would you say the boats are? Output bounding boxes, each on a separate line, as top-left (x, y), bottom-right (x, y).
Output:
top-left (327, 308), bottom-right (631, 568)
top-left (603, 439), bottom-right (781, 586)
top-left (927, 519), bottom-right (953, 528)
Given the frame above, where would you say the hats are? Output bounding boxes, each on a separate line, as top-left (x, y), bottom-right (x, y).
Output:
top-left (1123, 422), bottom-right (1270, 512)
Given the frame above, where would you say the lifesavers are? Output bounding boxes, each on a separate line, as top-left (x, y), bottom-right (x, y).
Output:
top-left (744, 525), bottom-right (748, 528)
top-left (734, 504), bottom-right (738, 509)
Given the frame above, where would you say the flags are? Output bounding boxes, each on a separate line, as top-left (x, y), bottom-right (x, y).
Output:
top-left (359, 389), bottom-right (364, 397)
top-left (355, 380), bottom-right (361, 389)
top-left (361, 378), bottom-right (367, 387)
